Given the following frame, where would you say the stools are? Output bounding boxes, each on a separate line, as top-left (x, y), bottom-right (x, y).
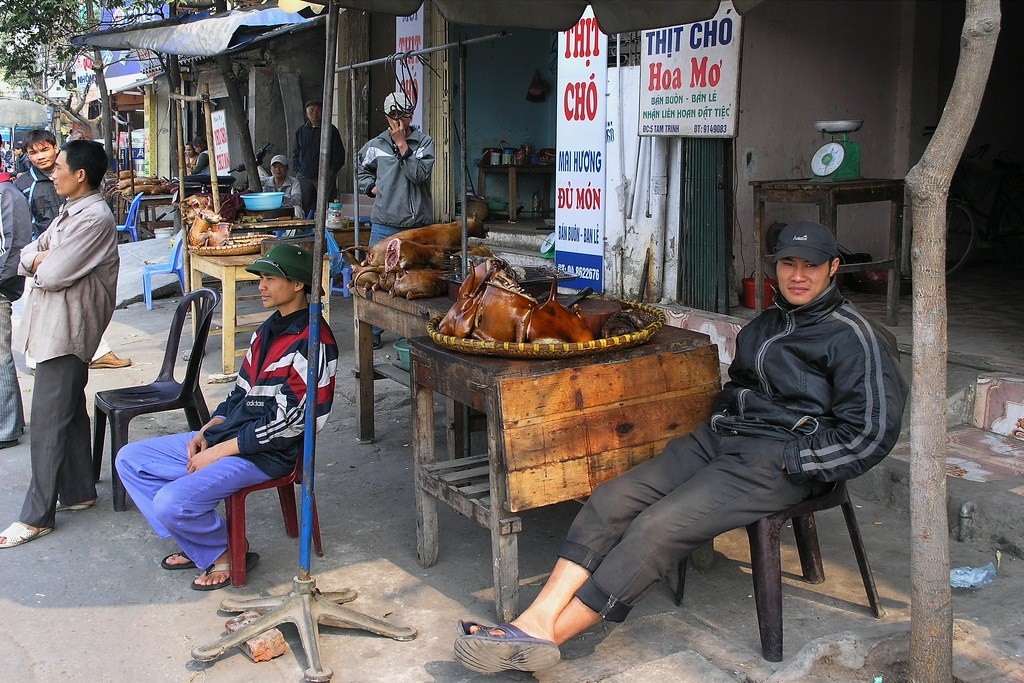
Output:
top-left (328, 262), bottom-right (353, 298)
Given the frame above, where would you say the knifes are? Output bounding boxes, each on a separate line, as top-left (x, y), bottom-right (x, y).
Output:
top-left (262, 216), bottom-right (291, 221)
top-left (562, 285), bottom-right (594, 310)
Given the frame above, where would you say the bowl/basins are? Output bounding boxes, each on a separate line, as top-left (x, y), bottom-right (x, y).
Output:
top-left (488, 201), bottom-right (509, 211)
top-left (239, 191), bottom-right (285, 211)
top-left (392, 338), bottom-right (410, 370)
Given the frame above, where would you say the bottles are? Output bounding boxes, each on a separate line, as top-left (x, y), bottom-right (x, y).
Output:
top-left (532, 187), bottom-right (541, 211)
top-left (516, 147), bottom-right (526, 167)
top-left (490, 149), bottom-right (501, 165)
top-left (502, 149), bottom-right (514, 165)
top-left (523, 136), bottom-right (533, 160)
top-left (327, 203), bottom-right (343, 229)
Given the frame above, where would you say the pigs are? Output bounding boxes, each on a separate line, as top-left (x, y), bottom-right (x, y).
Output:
top-left (113, 168), bottom-right (168, 196)
top-left (338, 210), bottom-right (499, 300)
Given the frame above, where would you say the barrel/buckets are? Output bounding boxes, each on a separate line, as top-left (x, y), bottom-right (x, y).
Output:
top-left (740, 277), bottom-right (776, 309)
top-left (539, 147), bottom-right (555, 161)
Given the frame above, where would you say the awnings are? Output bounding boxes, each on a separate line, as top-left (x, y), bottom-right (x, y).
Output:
top-left (138, 12), bottom-right (328, 76)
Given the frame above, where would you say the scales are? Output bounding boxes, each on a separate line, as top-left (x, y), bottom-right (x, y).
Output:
top-left (811, 121), bottom-right (864, 183)
top-left (539, 232), bottom-right (555, 259)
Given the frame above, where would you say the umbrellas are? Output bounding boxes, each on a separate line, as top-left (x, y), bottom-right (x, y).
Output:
top-left (301, 0), bottom-right (769, 35)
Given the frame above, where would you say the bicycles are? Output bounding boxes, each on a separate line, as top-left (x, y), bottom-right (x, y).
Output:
top-left (921, 125), bottom-right (1024, 277)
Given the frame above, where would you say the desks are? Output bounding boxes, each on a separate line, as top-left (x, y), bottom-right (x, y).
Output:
top-left (409, 319), bottom-right (726, 623)
top-left (116, 191), bottom-right (174, 246)
top-left (475, 162), bottom-right (555, 222)
top-left (189, 248), bottom-right (336, 376)
top-left (746, 176), bottom-right (906, 326)
top-left (352, 282), bottom-right (476, 518)
top-left (229, 217), bottom-right (316, 236)
top-left (325, 223), bottom-right (372, 250)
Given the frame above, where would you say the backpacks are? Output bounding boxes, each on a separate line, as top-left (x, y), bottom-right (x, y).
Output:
top-left (5, 150), bottom-right (12, 160)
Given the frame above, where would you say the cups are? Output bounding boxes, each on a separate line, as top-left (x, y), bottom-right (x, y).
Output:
top-left (343, 217), bottom-right (350, 229)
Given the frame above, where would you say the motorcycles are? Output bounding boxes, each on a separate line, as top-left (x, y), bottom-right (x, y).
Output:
top-left (164, 141), bottom-right (272, 233)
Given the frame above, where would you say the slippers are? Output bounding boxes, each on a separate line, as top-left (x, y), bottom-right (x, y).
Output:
top-left (161, 551), bottom-right (197, 569)
top-left (0, 521), bottom-right (53, 548)
top-left (455, 625), bottom-right (561, 673)
top-left (456, 619), bottom-right (495, 637)
top-left (56, 500), bottom-right (90, 511)
top-left (191, 553), bottom-right (260, 591)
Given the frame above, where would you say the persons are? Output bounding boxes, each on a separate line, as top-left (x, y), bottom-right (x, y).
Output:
top-left (184, 136), bottom-right (210, 176)
top-left (453, 221), bottom-right (908, 674)
top-left (293, 99), bottom-right (345, 221)
top-left (0, 126), bottom-right (119, 548)
top-left (262, 155), bottom-right (305, 237)
top-left (357, 92), bottom-right (434, 350)
top-left (113, 245), bottom-right (339, 591)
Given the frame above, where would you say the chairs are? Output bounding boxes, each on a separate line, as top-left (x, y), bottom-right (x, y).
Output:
top-left (228, 431), bottom-right (328, 588)
top-left (140, 226), bottom-right (188, 312)
top-left (671, 468), bottom-right (886, 663)
top-left (90, 285), bottom-right (223, 514)
top-left (114, 189), bottom-right (146, 242)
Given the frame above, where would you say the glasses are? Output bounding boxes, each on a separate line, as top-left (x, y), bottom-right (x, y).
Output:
top-left (79, 137), bottom-right (84, 139)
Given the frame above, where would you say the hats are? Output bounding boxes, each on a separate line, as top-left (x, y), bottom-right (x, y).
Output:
top-left (245, 244), bottom-right (326, 296)
top-left (194, 137), bottom-right (206, 144)
top-left (775, 221), bottom-right (837, 266)
top-left (305, 99), bottom-right (322, 107)
top-left (271, 155), bottom-right (289, 166)
top-left (384, 92), bottom-right (414, 114)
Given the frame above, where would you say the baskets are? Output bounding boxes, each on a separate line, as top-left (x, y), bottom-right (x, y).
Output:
top-left (188, 235), bottom-right (276, 255)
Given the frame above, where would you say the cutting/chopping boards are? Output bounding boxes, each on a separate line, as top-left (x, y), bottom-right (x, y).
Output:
top-left (539, 298), bottom-right (622, 340)
top-left (244, 206), bottom-right (295, 220)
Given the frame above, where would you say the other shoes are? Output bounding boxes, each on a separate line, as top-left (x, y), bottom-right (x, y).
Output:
top-left (90, 351), bottom-right (132, 369)
top-left (0, 439), bottom-right (19, 449)
